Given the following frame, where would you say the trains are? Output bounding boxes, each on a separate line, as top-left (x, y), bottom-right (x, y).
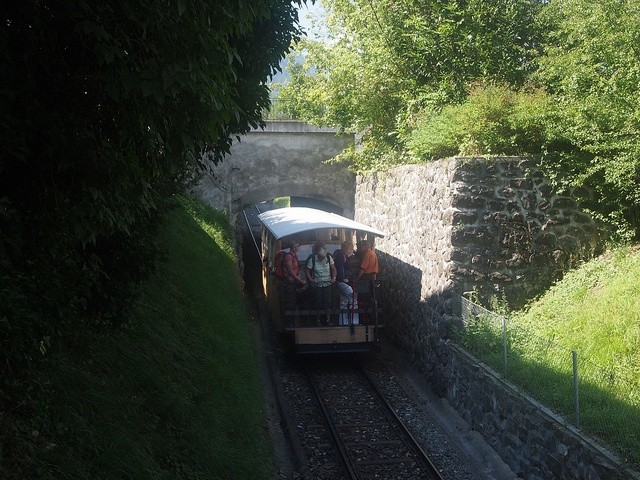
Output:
top-left (256, 204), bottom-right (387, 366)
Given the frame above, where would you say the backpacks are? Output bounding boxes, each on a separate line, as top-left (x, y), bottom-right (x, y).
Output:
top-left (275, 251), bottom-right (299, 280)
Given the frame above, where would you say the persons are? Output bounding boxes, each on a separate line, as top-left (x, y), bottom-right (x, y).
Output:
top-left (305, 241), bottom-right (337, 326)
top-left (275, 239), bottom-right (305, 323)
top-left (332, 241), bottom-right (359, 325)
top-left (353, 240), bottom-right (378, 325)
